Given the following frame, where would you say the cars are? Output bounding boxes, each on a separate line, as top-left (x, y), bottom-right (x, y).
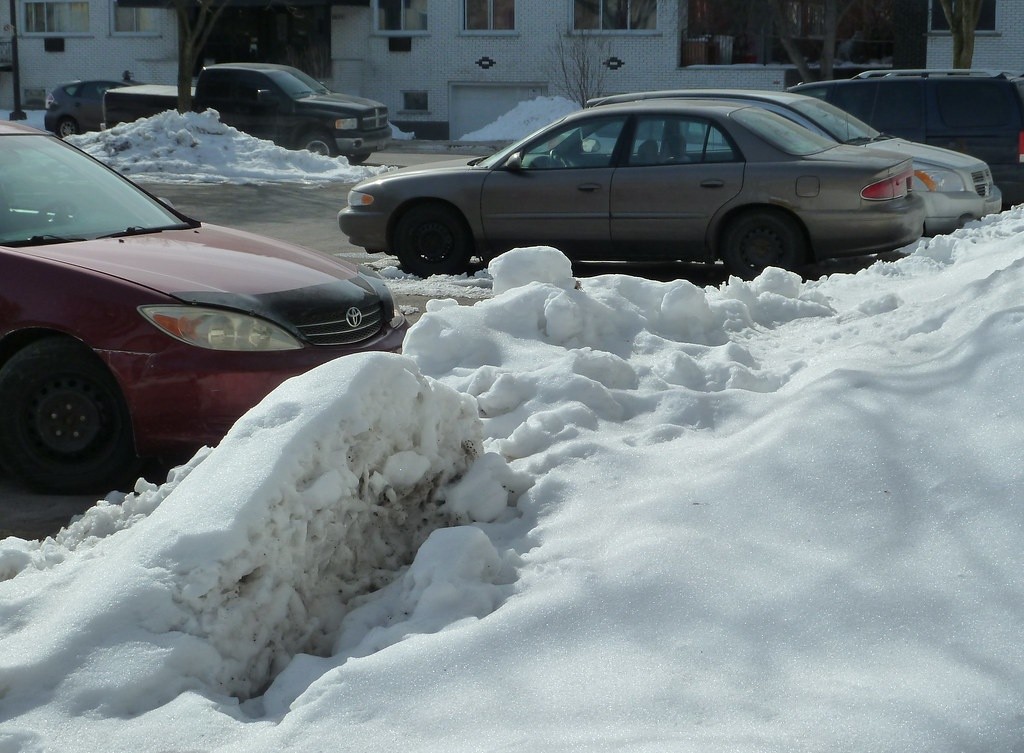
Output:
top-left (333, 97), bottom-right (928, 285)
top-left (43, 79), bottom-right (144, 140)
top-left (516, 89), bottom-right (1003, 239)
top-left (0, 119), bottom-right (410, 496)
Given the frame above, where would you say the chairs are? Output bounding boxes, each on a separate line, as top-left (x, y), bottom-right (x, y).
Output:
top-left (660, 134), bottom-right (690, 163)
top-left (636, 139), bottom-right (658, 164)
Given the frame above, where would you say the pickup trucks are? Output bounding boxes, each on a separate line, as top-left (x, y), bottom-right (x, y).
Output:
top-left (103, 62), bottom-right (393, 165)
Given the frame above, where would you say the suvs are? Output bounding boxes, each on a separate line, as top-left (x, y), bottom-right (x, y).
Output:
top-left (780, 66), bottom-right (1024, 217)
